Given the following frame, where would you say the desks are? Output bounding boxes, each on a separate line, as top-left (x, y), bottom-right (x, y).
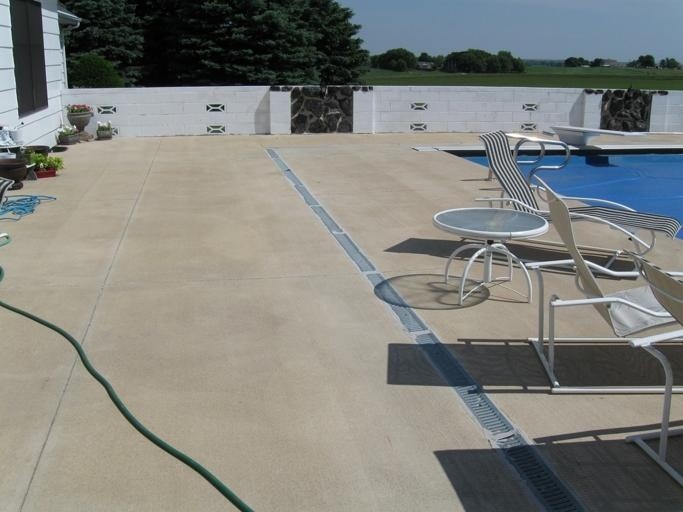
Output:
top-left (431, 206), bottom-right (550, 306)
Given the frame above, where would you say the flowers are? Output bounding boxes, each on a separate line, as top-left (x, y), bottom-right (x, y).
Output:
top-left (63, 103), bottom-right (92, 113)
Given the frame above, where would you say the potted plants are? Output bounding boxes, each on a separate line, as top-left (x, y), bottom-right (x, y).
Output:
top-left (57, 126), bottom-right (81, 145)
top-left (34, 153), bottom-right (65, 178)
top-left (96, 121), bottom-right (112, 139)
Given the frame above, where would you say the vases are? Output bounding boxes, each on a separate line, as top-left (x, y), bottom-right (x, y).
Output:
top-left (67, 113), bottom-right (91, 141)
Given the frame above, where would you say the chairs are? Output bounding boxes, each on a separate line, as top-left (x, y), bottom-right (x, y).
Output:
top-left (474, 132), bottom-right (683, 258)
top-left (528, 177), bottom-right (682, 397)
top-left (625, 245), bottom-right (681, 485)
